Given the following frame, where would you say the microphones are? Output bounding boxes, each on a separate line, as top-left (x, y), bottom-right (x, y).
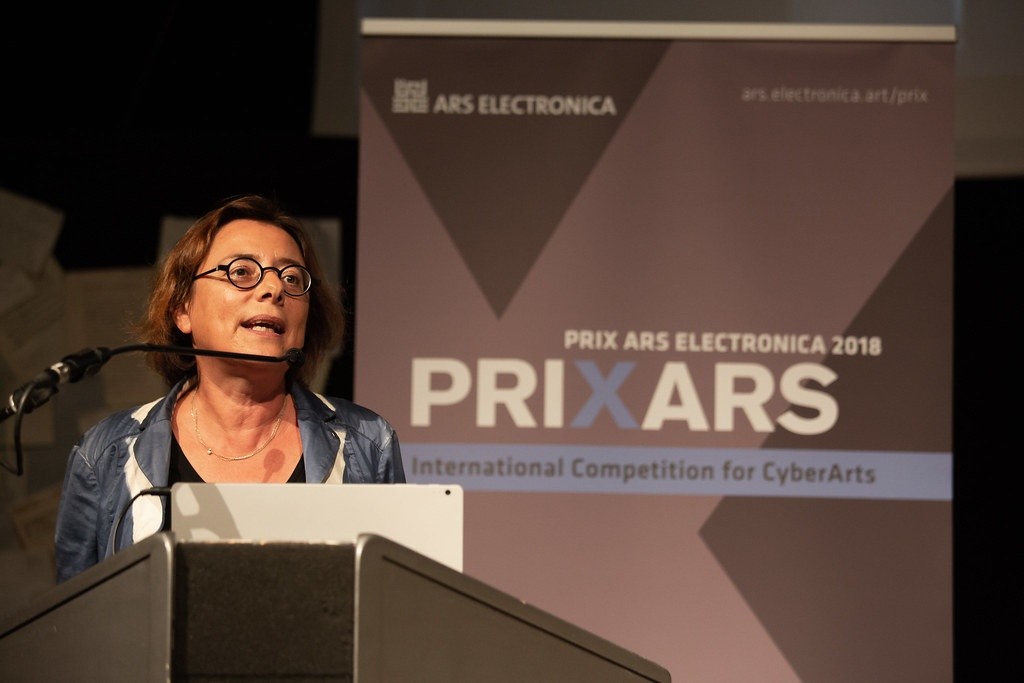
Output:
top-left (33, 346), bottom-right (306, 390)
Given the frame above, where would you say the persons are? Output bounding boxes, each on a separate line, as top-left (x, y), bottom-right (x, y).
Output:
top-left (51, 195), bottom-right (408, 583)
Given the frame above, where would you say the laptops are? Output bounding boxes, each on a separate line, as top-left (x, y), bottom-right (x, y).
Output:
top-left (169, 482), bottom-right (464, 578)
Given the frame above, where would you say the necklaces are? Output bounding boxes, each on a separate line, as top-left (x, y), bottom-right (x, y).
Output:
top-left (190, 384), bottom-right (287, 461)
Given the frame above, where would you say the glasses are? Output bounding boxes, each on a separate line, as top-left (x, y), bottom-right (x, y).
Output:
top-left (192, 257), bottom-right (321, 297)
